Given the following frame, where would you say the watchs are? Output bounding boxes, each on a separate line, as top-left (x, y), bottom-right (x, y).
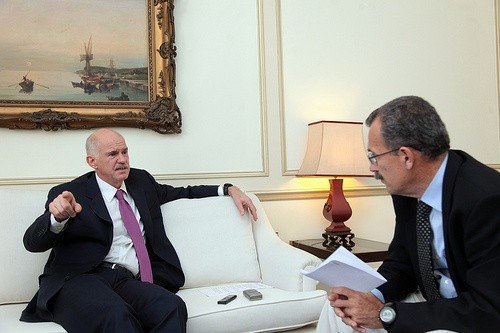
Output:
top-left (378, 303), bottom-right (396, 331)
top-left (223, 183), bottom-right (232, 197)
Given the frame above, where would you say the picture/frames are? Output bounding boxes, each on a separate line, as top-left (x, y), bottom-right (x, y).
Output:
top-left (0, 0), bottom-right (182, 135)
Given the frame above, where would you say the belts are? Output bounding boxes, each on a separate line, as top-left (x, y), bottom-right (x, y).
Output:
top-left (100, 261), bottom-right (131, 275)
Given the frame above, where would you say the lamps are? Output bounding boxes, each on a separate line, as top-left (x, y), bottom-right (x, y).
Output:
top-left (295, 120), bottom-right (375, 252)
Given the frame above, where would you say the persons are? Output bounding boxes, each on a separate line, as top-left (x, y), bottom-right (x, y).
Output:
top-left (328, 96), bottom-right (500, 333)
top-left (19, 129), bottom-right (257, 333)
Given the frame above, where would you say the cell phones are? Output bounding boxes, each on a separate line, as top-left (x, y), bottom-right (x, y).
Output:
top-left (243, 288), bottom-right (263, 301)
top-left (218, 294), bottom-right (237, 305)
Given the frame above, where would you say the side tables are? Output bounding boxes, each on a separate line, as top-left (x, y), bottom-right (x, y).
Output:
top-left (290, 237), bottom-right (391, 262)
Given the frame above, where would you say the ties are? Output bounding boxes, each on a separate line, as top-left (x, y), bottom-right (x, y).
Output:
top-left (416, 201), bottom-right (442, 303)
top-left (114, 190), bottom-right (153, 284)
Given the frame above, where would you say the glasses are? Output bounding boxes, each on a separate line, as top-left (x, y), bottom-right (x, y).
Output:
top-left (367, 147), bottom-right (400, 165)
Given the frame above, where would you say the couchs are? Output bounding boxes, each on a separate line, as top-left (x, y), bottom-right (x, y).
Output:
top-left (0, 187), bottom-right (328, 333)
